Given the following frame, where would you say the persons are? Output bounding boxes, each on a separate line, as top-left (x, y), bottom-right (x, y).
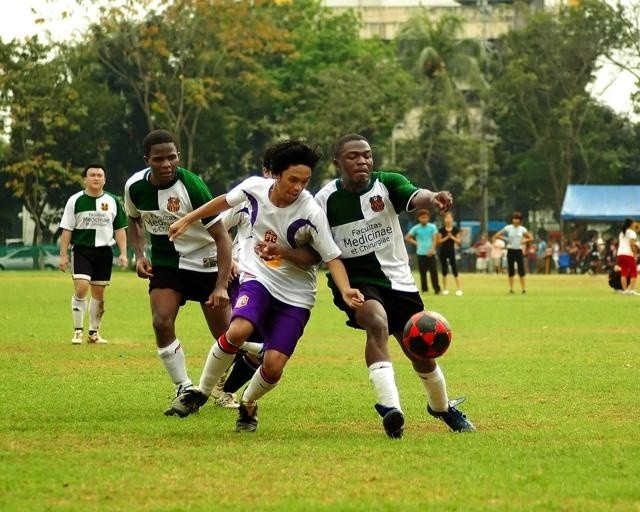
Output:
top-left (471, 211), bottom-right (640, 297)
top-left (59, 164), bottom-right (128, 344)
top-left (405, 208), bottom-right (443, 295)
top-left (437, 212), bottom-right (463, 296)
top-left (168, 138), bottom-right (364, 433)
top-left (254, 133), bottom-right (476, 438)
top-left (209, 144), bottom-right (276, 409)
top-left (123, 129), bottom-right (231, 414)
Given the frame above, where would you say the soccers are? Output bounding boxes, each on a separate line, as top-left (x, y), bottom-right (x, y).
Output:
top-left (403, 311), bottom-right (452, 360)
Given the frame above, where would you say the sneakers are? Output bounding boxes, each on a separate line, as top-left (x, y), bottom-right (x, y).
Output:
top-left (373, 403), bottom-right (405, 439)
top-left (71, 329), bottom-right (84, 345)
top-left (163, 371), bottom-right (259, 434)
top-left (87, 332), bottom-right (108, 344)
top-left (426, 396), bottom-right (477, 434)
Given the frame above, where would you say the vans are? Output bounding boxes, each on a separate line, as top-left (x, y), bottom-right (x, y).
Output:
top-left (3, 238), bottom-right (24, 254)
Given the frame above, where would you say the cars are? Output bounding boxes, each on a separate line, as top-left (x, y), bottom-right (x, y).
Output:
top-left (112, 250), bottom-right (146, 269)
top-left (0, 248), bottom-right (68, 273)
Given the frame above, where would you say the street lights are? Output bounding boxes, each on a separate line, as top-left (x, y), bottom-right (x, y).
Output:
top-left (391, 121), bottom-right (404, 165)
